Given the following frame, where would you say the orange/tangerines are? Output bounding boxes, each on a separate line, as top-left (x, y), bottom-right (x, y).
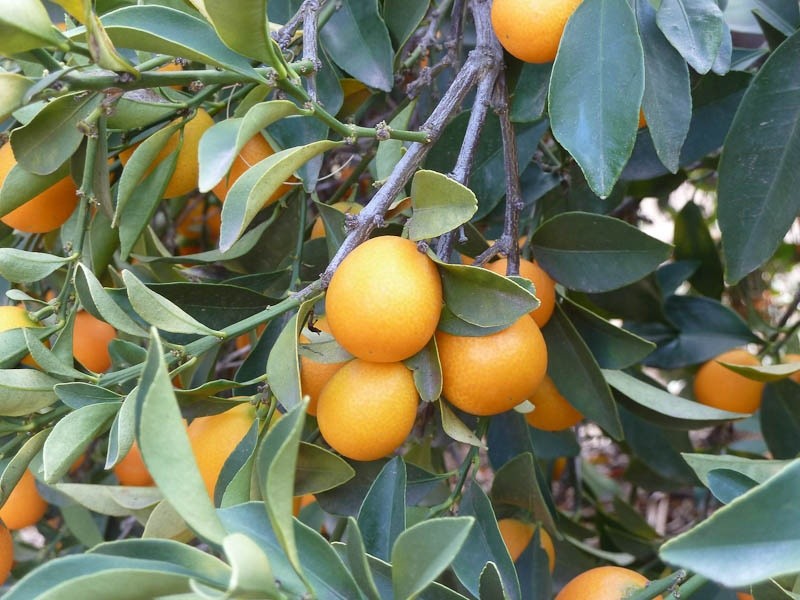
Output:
top-left (0, 0), bottom-right (799, 600)
